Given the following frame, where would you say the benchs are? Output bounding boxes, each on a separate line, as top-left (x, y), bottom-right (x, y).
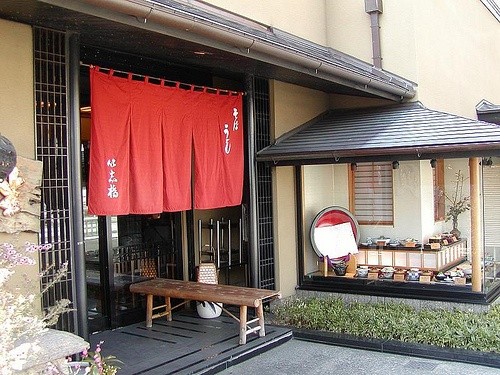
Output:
top-left (86, 270), bottom-right (155, 329)
top-left (129, 277), bottom-right (282, 344)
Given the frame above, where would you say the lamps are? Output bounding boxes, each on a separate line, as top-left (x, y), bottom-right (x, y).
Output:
top-left (350, 157), bottom-right (494, 171)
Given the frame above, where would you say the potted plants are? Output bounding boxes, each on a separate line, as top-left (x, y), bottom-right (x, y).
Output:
top-left (436, 170), bottom-right (472, 238)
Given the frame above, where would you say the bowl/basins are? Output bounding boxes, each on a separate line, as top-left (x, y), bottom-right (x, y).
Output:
top-left (373, 237), bottom-right (390, 244)
top-left (311, 205), bottom-right (361, 262)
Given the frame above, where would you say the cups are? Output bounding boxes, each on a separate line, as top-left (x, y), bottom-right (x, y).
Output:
top-left (361, 242), bottom-right (368, 246)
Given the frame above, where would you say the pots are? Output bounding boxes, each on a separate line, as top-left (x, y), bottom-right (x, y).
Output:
top-left (379, 267), bottom-right (395, 278)
top-left (331, 263), bottom-right (349, 276)
top-left (353, 267), bottom-right (369, 278)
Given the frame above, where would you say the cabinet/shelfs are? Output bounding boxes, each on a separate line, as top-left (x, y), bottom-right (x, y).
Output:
top-left (198, 217), bottom-right (242, 272)
